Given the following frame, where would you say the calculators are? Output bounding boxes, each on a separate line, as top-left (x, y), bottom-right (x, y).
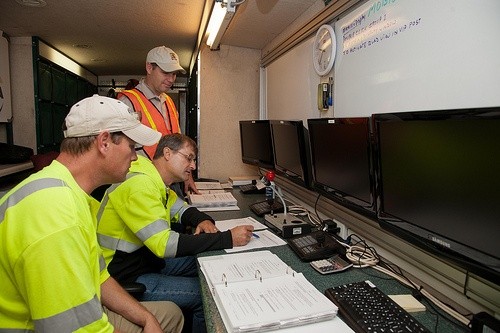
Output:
top-left (309, 253), bottom-right (353, 275)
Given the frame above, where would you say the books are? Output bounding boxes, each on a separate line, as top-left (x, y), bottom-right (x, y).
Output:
top-left (186, 190), bottom-right (240, 212)
top-left (229, 176), bottom-right (262, 186)
top-left (196, 250), bottom-right (339, 333)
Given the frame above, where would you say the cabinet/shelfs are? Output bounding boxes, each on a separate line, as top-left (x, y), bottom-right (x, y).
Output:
top-left (11, 35), bottom-right (99, 156)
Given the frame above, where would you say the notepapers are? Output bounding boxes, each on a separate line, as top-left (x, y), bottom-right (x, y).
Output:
top-left (387, 294), bottom-right (426, 313)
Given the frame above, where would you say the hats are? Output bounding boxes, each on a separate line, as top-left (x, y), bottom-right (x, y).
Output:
top-left (146, 45), bottom-right (186, 74)
top-left (63, 93), bottom-right (163, 146)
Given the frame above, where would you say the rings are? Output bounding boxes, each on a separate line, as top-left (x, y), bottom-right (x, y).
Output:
top-left (214, 229), bottom-right (216, 230)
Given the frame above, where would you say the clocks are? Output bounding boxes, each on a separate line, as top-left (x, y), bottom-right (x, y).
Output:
top-left (312, 24), bottom-right (336, 76)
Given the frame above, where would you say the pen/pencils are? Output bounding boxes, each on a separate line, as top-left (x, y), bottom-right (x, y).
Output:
top-left (252, 233), bottom-right (259, 239)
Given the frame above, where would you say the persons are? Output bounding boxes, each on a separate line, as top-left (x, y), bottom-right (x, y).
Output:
top-left (116, 46), bottom-right (202, 201)
top-left (96, 132), bottom-right (254, 333)
top-left (0, 94), bottom-right (184, 333)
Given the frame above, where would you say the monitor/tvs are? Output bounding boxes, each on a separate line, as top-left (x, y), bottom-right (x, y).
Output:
top-left (238, 104), bottom-right (500, 286)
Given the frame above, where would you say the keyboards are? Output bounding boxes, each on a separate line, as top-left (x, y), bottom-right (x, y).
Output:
top-left (323, 279), bottom-right (432, 333)
top-left (250, 199), bottom-right (289, 216)
top-left (288, 231), bottom-right (343, 262)
top-left (239, 184), bottom-right (267, 194)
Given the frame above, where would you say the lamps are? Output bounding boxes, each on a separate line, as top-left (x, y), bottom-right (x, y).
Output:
top-left (206, 0), bottom-right (245, 50)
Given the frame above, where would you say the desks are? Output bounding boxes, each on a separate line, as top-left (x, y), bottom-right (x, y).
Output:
top-left (195, 186), bottom-right (476, 333)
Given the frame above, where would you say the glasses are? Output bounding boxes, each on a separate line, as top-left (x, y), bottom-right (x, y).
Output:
top-left (161, 146), bottom-right (196, 163)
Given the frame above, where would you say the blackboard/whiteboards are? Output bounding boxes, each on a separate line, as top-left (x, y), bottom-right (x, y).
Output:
top-left (333, 0), bottom-right (500, 118)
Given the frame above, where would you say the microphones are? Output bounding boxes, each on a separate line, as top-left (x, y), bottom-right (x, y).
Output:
top-left (259, 175), bottom-right (288, 215)
